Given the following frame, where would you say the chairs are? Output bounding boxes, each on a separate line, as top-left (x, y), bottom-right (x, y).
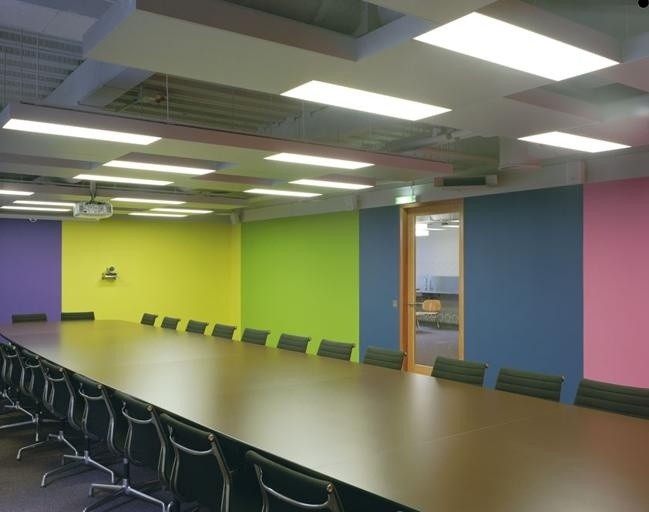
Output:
top-left (416, 299), bottom-right (442, 328)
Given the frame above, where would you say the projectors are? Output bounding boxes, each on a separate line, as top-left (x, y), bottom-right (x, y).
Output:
top-left (73, 203), bottom-right (113, 220)
